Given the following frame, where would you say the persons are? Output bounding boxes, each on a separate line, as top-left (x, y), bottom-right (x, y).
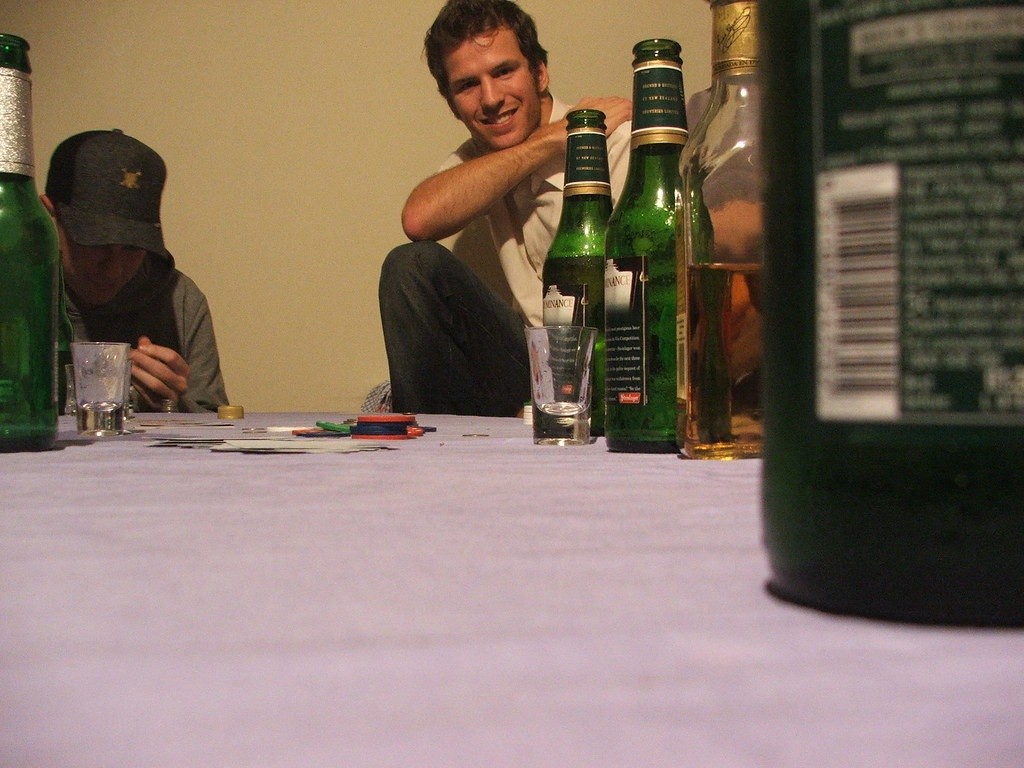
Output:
top-left (37, 127), bottom-right (231, 414)
top-left (375, 0), bottom-right (637, 421)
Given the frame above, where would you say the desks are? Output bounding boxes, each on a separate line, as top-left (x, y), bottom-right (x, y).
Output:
top-left (0, 414), bottom-right (1024, 768)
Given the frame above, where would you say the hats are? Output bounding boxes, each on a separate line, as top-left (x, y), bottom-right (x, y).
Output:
top-left (46, 129), bottom-right (167, 254)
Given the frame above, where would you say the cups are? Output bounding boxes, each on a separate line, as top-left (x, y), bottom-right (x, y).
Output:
top-left (71, 341), bottom-right (130, 438)
top-left (525, 326), bottom-right (598, 446)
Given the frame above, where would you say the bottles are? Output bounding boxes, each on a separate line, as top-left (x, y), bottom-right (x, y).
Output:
top-left (0, 33), bottom-right (61, 452)
top-left (602, 39), bottom-right (692, 455)
top-left (543, 109), bottom-right (615, 437)
top-left (762, 0), bottom-right (1024, 629)
top-left (683, 0), bottom-right (770, 461)
